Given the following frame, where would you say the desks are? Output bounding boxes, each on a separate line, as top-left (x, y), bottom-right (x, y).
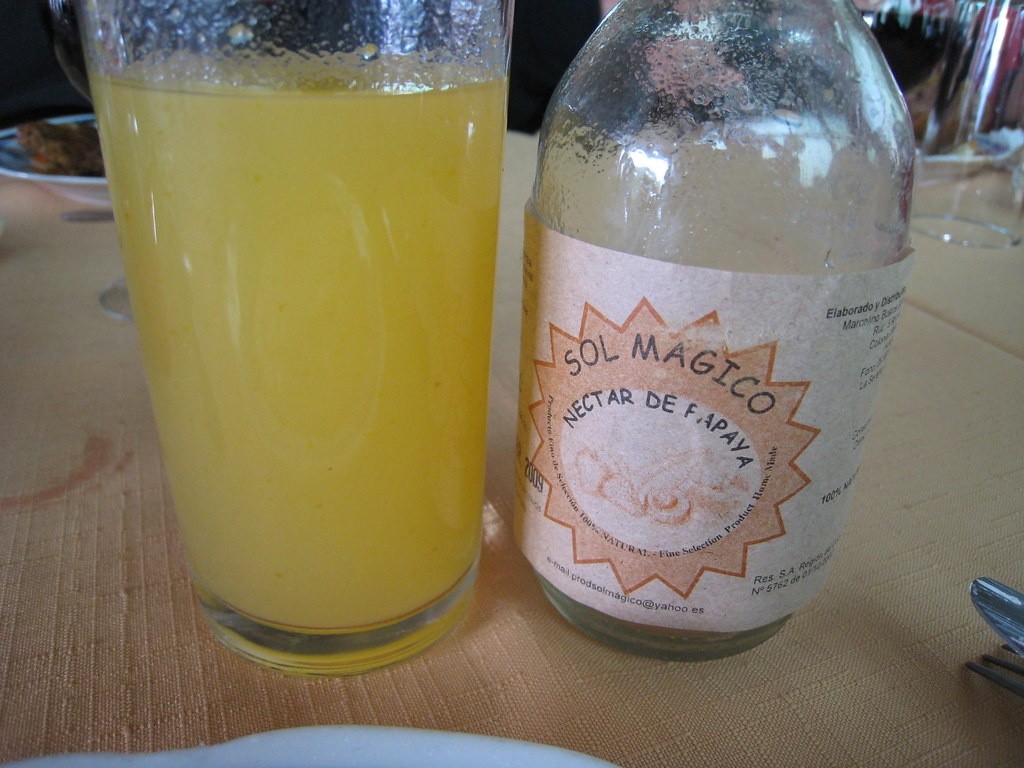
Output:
top-left (0, 128), bottom-right (1024, 768)
top-left (608, 137), bottom-right (1024, 360)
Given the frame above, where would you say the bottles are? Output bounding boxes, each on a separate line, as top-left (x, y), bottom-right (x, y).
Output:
top-left (525, 0), bottom-right (922, 658)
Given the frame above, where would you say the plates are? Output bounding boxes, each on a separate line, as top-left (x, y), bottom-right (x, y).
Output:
top-left (1, 114), bottom-right (110, 207)
top-left (1, 724), bottom-right (639, 768)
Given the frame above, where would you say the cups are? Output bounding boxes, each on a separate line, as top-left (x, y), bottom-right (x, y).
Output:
top-left (908, 1), bottom-right (1024, 252)
top-left (68, 1), bottom-right (515, 676)
